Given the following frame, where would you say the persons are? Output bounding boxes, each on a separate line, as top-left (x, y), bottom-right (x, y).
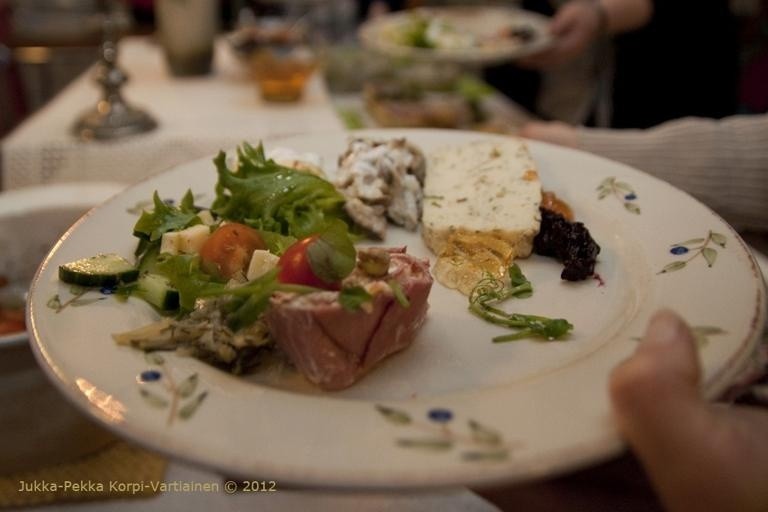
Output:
top-left (480, 91), bottom-right (767, 233)
top-left (364, 1), bottom-right (768, 130)
top-left (607, 307), bottom-right (767, 511)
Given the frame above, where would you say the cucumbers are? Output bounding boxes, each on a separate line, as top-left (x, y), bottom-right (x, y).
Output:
top-left (135, 275), bottom-right (181, 312)
top-left (59, 252), bottom-right (138, 287)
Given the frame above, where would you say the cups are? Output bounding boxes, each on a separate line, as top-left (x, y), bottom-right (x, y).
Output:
top-left (158, 2), bottom-right (216, 78)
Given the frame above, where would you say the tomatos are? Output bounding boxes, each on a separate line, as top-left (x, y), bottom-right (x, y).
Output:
top-left (277, 232), bottom-right (343, 290)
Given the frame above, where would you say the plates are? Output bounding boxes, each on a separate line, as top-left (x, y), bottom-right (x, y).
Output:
top-left (25, 124), bottom-right (766, 490)
top-left (356, 5), bottom-right (559, 62)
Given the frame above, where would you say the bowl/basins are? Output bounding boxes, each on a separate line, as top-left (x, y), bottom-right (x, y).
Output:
top-left (226, 24), bottom-right (331, 104)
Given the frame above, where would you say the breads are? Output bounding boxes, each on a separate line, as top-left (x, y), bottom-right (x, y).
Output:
top-left (260, 246), bottom-right (434, 391)
top-left (420, 138), bottom-right (544, 259)
